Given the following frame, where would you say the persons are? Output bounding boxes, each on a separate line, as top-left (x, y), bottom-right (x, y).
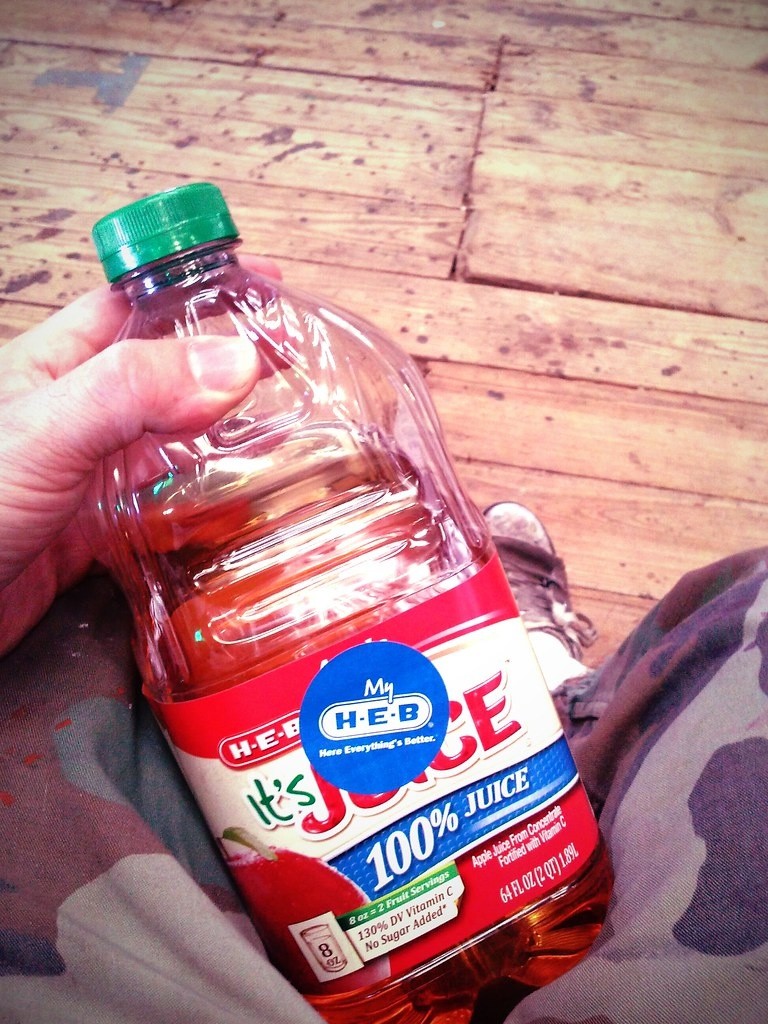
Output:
top-left (0, 255), bottom-right (768, 1024)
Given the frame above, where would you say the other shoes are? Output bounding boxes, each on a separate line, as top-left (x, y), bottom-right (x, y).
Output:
top-left (482, 501), bottom-right (579, 658)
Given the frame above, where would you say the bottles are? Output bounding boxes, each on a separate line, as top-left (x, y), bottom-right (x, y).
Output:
top-left (92, 182), bottom-right (616, 1024)
